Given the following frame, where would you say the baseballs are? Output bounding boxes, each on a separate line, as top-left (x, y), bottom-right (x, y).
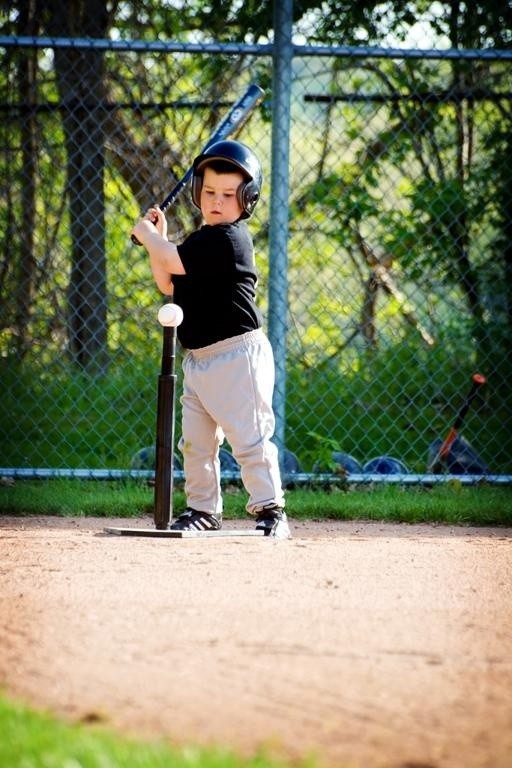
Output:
top-left (157, 304), bottom-right (183, 328)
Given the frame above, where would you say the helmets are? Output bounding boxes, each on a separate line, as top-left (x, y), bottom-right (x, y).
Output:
top-left (130, 445), bottom-right (411, 478)
top-left (191, 141), bottom-right (264, 220)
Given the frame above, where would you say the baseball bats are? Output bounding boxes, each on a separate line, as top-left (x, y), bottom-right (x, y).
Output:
top-left (430, 374), bottom-right (485, 476)
top-left (130, 85), bottom-right (266, 246)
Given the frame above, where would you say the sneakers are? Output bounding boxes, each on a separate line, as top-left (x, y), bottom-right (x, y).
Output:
top-left (169, 506), bottom-right (222, 531)
top-left (255, 505), bottom-right (291, 537)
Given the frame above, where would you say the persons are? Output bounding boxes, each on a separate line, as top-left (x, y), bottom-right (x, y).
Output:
top-left (131, 141), bottom-right (290, 542)
